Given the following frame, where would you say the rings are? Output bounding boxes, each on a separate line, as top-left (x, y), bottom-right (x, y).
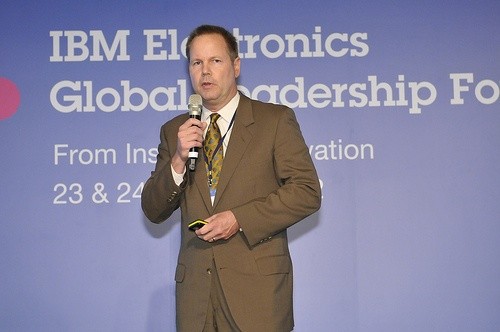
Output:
top-left (212, 238), bottom-right (216, 242)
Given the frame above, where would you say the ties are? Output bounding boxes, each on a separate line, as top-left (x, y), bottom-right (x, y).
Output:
top-left (203, 113), bottom-right (224, 208)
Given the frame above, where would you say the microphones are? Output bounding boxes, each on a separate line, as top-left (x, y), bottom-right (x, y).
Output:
top-left (187, 93), bottom-right (202, 170)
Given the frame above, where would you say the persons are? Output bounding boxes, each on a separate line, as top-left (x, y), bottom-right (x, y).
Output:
top-left (140, 24), bottom-right (323, 332)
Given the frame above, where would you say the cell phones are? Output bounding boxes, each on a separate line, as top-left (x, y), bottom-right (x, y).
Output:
top-left (188, 219), bottom-right (208, 231)
